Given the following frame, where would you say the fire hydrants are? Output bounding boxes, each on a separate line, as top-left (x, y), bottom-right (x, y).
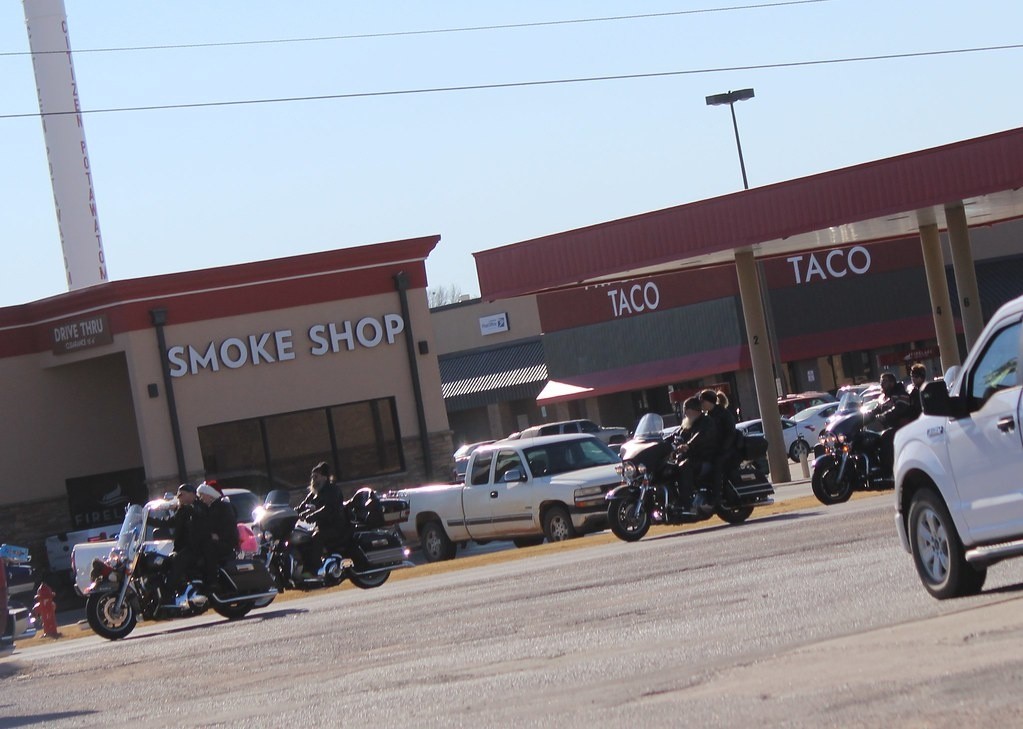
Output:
top-left (31, 584), bottom-right (62, 637)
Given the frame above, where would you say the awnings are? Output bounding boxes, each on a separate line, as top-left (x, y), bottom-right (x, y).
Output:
top-left (780, 316), bottom-right (964, 362)
top-left (433, 340), bottom-right (548, 414)
top-left (536, 345), bottom-right (752, 406)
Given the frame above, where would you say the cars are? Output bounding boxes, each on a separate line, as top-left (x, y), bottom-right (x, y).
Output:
top-left (791, 401), bottom-right (862, 439)
top-left (777, 392), bottom-right (839, 416)
top-left (735, 417), bottom-right (820, 470)
top-left (836, 377), bottom-right (914, 403)
top-left (892, 296), bottom-right (1022, 601)
top-left (453, 431), bottom-right (522, 476)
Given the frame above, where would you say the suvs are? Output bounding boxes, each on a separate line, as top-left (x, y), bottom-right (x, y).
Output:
top-left (508, 419), bottom-right (629, 453)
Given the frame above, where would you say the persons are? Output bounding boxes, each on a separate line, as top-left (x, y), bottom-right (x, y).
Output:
top-left (664, 389), bottom-right (735, 498)
top-left (864, 363), bottom-right (928, 425)
top-left (294, 462), bottom-right (343, 554)
top-left (133, 480), bottom-right (237, 593)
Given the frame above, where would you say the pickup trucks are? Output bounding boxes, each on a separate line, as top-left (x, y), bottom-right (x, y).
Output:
top-left (69, 488), bottom-right (266, 614)
top-left (396, 434), bottom-right (631, 563)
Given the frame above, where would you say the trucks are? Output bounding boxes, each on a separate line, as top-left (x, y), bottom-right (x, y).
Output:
top-left (45, 466), bottom-right (307, 607)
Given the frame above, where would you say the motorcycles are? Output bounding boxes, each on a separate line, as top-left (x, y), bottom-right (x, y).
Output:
top-left (604, 411), bottom-right (776, 542)
top-left (248, 488), bottom-right (416, 611)
top-left (80, 502), bottom-right (281, 640)
top-left (810, 391), bottom-right (894, 506)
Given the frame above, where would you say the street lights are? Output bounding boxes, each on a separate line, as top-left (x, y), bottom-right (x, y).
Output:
top-left (704, 86), bottom-right (755, 191)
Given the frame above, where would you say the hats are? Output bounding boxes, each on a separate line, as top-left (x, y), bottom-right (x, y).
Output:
top-left (175, 483), bottom-right (197, 499)
top-left (682, 398), bottom-right (701, 411)
top-left (162, 492), bottom-right (174, 499)
top-left (311, 462), bottom-right (335, 480)
top-left (195, 478), bottom-right (230, 502)
